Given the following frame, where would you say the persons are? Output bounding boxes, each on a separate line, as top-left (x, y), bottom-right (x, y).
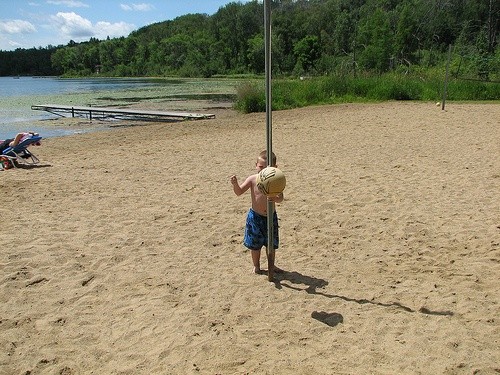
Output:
top-left (0, 133), bottom-right (41, 155)
top-left (230, 150), bottom-right (286, 274)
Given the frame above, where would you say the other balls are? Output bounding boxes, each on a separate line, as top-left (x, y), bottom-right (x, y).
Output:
top-left (257, 167), bottom-right (287, 197)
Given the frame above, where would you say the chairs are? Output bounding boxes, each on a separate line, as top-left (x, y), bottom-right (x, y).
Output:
top-left (0, 136), bottom-right (42, 168)
